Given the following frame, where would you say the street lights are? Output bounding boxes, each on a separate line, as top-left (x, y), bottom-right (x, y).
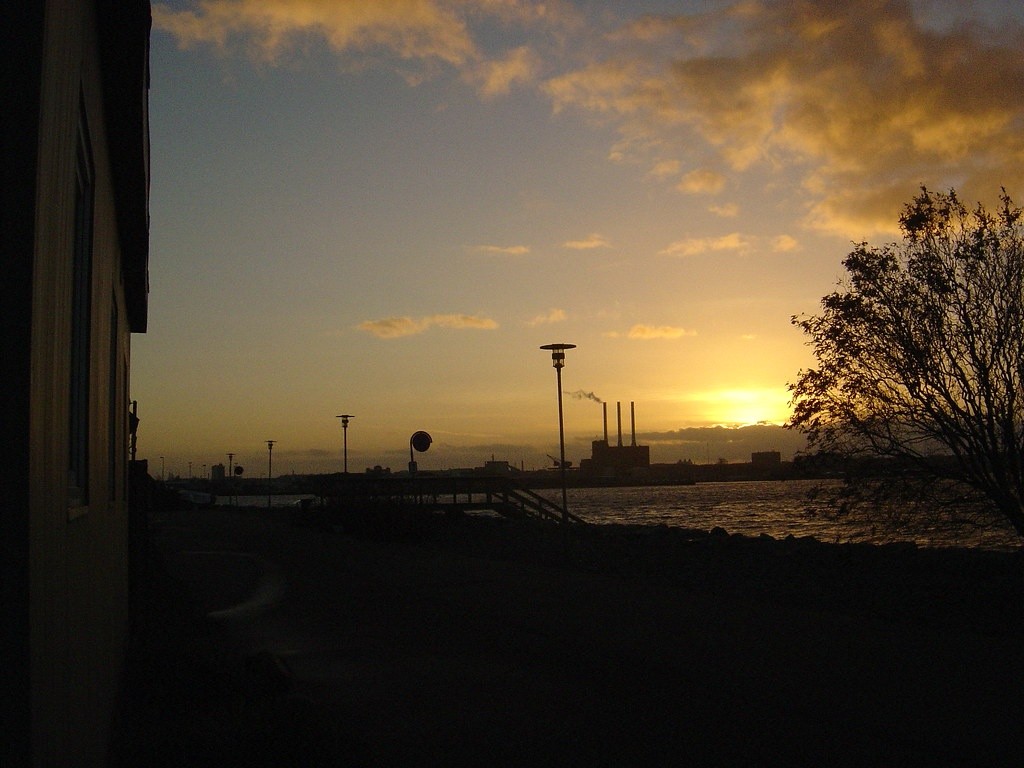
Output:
top-left (538, 343), bottom-right (578, 521)
top-left (160, 456), bottom-right (165, 482)
top-left (226, 453), bottom-right (235, 504)
top-left (267, 440), bottom-right (278, 518)
top-left (336, 413), bottom-right (355, 471)
